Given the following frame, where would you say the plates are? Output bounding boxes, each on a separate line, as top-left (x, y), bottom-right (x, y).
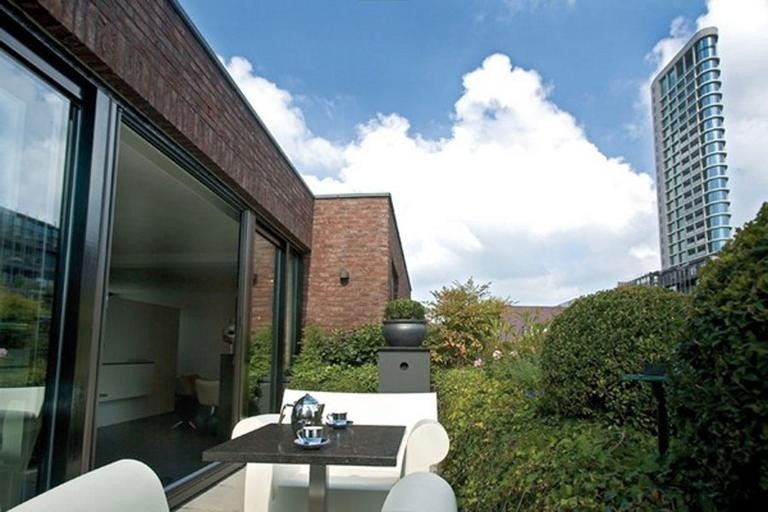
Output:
top-left (293, 437), bottom-right (331, 450)
top-left (326, 421), bottom-right (353, 429)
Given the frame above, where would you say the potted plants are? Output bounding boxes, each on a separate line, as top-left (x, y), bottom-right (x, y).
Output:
top-left (380, 296), bottom-right (429, 347)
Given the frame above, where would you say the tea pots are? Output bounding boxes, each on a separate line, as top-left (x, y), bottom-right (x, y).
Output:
top-left (278, 393), bottom-right (325, 434)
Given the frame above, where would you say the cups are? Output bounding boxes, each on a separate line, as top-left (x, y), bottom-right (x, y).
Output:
top-left (326, 412), bottom-right (347, 425)
top-left (296, 426), bottom-right (323, 444)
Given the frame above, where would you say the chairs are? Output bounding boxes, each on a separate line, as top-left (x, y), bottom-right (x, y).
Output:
top-left (0, 386), bottom-right (47, 512)
top-left (194, 377), bottom-right (220, 433)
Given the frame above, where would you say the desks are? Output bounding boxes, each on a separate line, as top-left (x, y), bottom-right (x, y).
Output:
top-left (202, 421), bottom-right (406, 511)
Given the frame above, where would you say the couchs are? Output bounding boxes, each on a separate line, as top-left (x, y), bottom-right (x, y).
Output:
top-left (231, 388), bottom-right (451, 512)
